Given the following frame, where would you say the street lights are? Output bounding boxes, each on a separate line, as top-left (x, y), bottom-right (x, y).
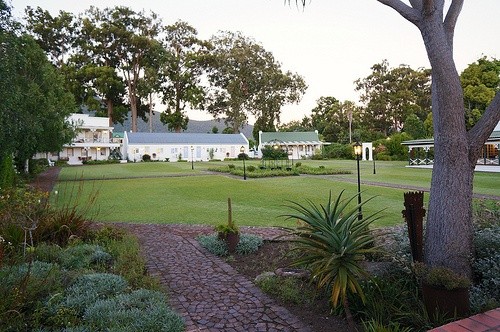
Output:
top-left (353, 141), bottom-right (363, 223)
top-left (240, 145), bottom-right (246, 180)
top-left (190, 145), bottom-right (194, 169)
